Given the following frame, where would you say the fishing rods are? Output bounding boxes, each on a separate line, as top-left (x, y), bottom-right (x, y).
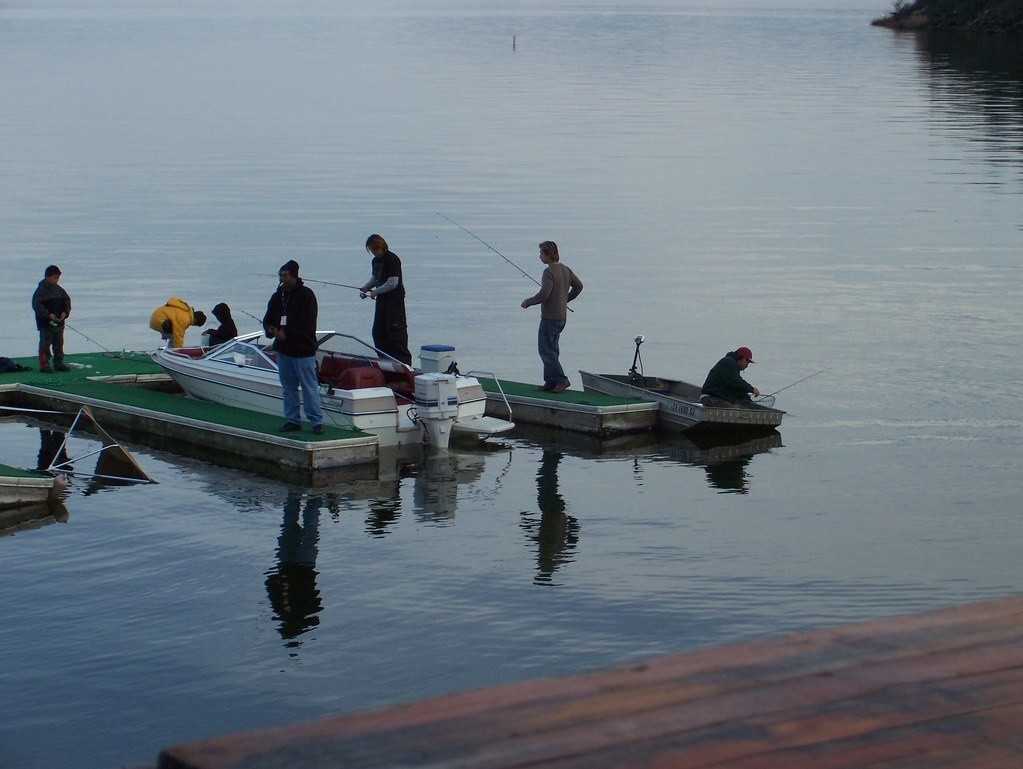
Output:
top-left (234, 309), bottom-right (266, 323)
top-left (57, 317), bottom-right (121, 361)
top-left (436, 211), bottom-right (576, 313)
top-left (754, 367), bottom-right (830, 399)
top-left (249, 272), bottom-right (373, 292)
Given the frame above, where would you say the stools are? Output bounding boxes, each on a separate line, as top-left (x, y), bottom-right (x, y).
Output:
top-left (419, 344), bottom-right (456, 373)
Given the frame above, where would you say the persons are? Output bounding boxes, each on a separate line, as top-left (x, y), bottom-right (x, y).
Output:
top-left (521, 241), bottom-right (584, 394)
top-left (360, 234), bottom-right (412, 368)
top-left (263, 260), bottom-right (324, 434)
top-left (32, 265), bottom-right (71, 374)
top-left (701, 347), bottom-right (759, 410)
top-left (202, 302), bottom-right (238, 346)
top-left (150, 298), bottom-right (206, 348)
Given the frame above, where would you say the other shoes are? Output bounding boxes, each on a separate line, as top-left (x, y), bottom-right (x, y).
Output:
top-left (551, 376), bottom-right (570, 392)
top-left (538, 382), bottom-right (556, 391)
top-left (278, 421), bottom-right (302, 433)
top-left (53, 366), bottom-right (71, 371)
top-left (40, 367), bottom-right (54, 373)
top-left (313, 424), bottom-right (324, 435)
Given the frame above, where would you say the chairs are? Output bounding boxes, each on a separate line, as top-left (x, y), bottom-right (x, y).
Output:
top-left (336, 368), bottom-right (385, 389)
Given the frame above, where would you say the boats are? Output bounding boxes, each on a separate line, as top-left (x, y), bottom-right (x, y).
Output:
top-left (150, 327), bottom-right (515, 450)
top-left (577, 332), bottom-right (788, 433)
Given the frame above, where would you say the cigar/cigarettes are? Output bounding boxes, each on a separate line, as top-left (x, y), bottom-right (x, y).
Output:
top-left (279, 282), bottom-right (283, 287)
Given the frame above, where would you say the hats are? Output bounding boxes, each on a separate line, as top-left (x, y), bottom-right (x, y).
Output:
top-left (736, 346), bottom-right (754, 363)
top-left (280, 260), bottom-right (299, 277)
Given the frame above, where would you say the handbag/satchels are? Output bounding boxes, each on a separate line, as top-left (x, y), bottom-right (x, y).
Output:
top-left (0, 357), bottom-right (32, 372)
